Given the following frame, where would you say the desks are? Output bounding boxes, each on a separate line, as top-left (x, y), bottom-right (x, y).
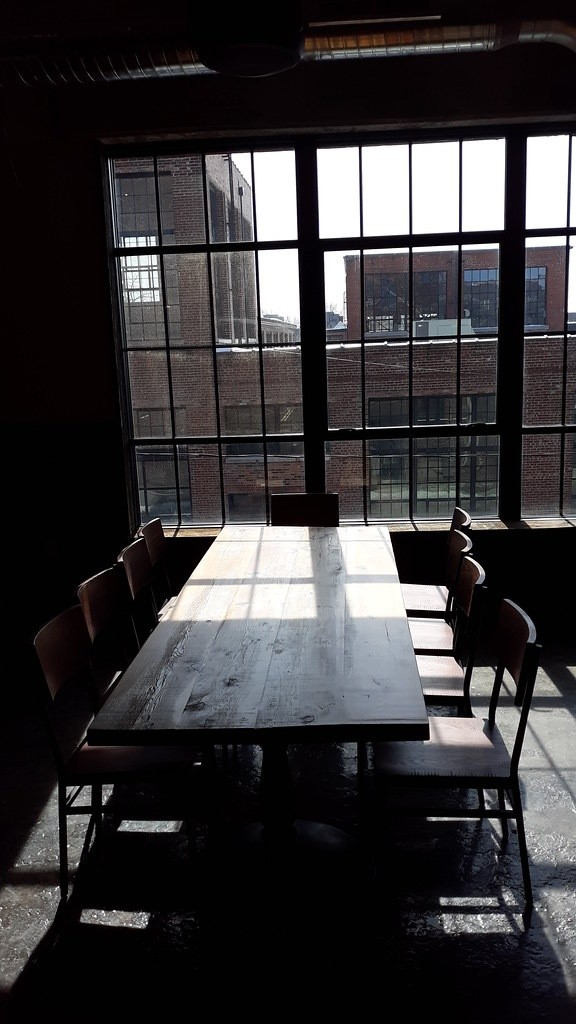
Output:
top-left (86, 520), bottom-right (429, 897)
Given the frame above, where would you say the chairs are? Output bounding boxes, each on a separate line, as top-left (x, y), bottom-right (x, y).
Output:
top-left (30, 492), bottom-right (539, 946)
